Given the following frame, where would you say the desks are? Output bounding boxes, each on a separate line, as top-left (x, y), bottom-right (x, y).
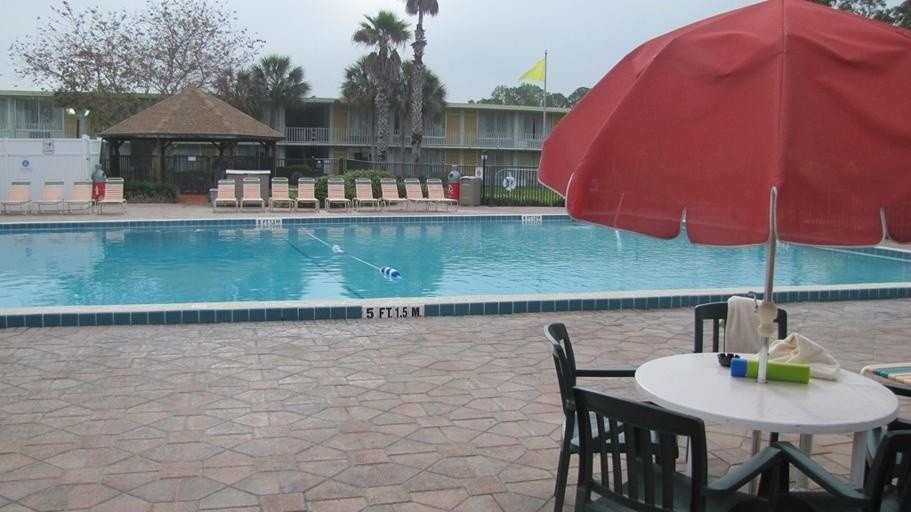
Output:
top-left (633, 351), bottom-right (901, 448)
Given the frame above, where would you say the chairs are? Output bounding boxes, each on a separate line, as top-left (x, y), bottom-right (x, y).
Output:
top-left (571, 386), bottom-right (770, 510)
top-left (543, 321), bottom-right (668, 511)
top-left (207, 175), bottom-right (461, 216)
top-left (688, 301), bottom-right (789, 356)
top-left (774, 359), bottom-right (911, 512)
top-left (0, 178), bottom-right (130, 215)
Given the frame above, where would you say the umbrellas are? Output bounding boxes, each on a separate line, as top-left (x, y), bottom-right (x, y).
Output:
top-left (535, 0), bottom-right (911, 495)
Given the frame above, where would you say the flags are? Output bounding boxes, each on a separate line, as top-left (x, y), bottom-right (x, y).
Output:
top-left (517, 57), bottom-right (545, 83)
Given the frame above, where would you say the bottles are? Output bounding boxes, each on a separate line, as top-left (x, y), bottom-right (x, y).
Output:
top-left (730, 357), bottom-right (811, 384)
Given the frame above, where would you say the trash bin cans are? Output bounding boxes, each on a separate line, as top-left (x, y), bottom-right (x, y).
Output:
top-left (448, 165), bottom-right (481, 206)
top-left (92, 163), bottom-right (106, 201)
